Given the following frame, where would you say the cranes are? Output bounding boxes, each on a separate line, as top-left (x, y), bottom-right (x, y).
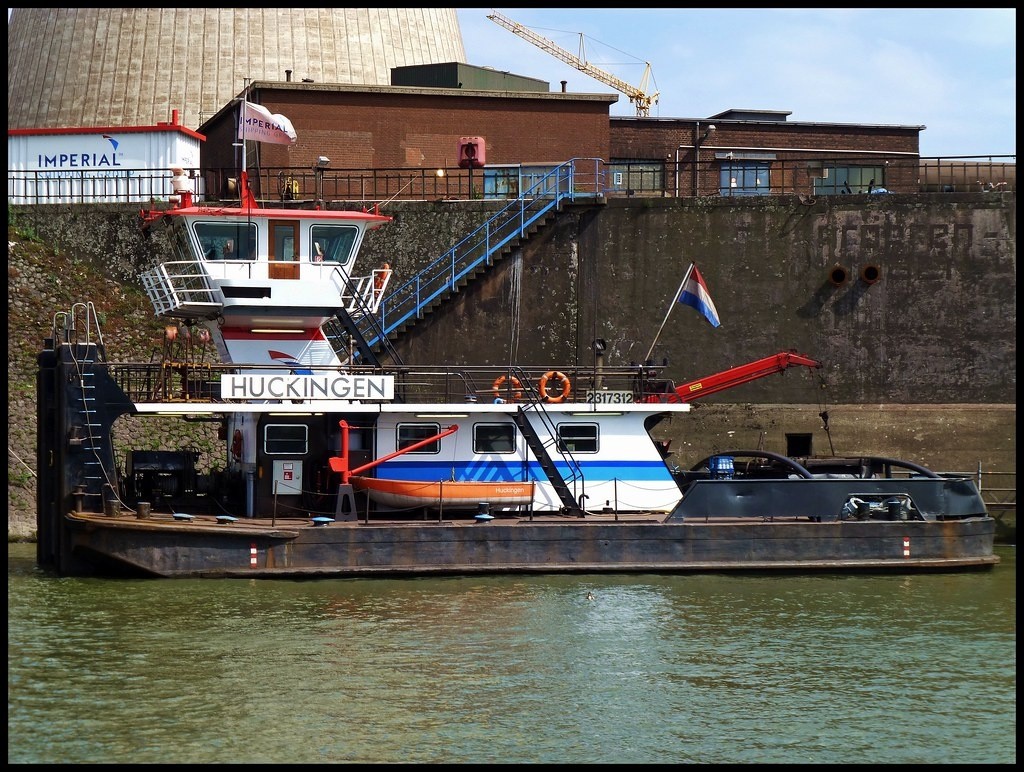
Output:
top-left (484, 8), bottom-right (663, 118)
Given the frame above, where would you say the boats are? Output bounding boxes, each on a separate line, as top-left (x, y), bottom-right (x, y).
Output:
top-left (55, 63), bottom-right (1005, 573)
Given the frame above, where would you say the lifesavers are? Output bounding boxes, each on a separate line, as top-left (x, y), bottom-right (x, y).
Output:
top-left (373, 262), bottom-right (390, 290)
top-left (492, 374), bottom-right (523, 404)
top-left (539, 370), bottom-right (571, 404)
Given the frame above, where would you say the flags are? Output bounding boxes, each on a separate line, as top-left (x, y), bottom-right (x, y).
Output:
top-left (236, 100), bottom-right (297, 147)
top-left (675, 265), bottom-right (722, 328)
